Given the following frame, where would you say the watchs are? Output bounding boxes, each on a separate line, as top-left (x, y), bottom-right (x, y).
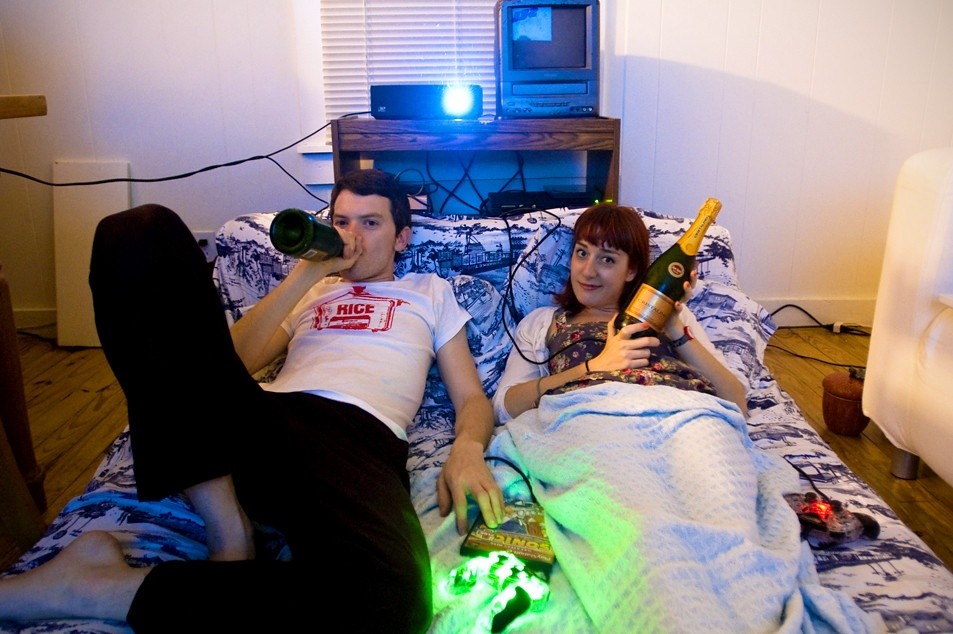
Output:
top-left (668, 325), bottom-right (695, 347)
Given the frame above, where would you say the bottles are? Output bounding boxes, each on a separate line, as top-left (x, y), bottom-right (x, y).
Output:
top-left (613, 197), bottom-right (722, 339)
top-left (268, 207), bottom-right (345, 262)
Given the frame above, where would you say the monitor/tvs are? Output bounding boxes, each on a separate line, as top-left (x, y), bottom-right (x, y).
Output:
top-left (494, 0), bottom-right (601, 118)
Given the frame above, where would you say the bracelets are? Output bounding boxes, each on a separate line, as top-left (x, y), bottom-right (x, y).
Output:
top-left (584, 360), bottom-right (591, 372)
top-left (536, 377), bottom-right (544, 396)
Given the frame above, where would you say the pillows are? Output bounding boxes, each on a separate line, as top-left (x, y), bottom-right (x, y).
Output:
top-left (498, 216), bottom-right (780, 401)
top-left (406, 274), bottom-right (518, 432)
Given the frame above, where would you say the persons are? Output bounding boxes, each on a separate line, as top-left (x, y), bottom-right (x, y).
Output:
top-left (492, 202), bottom-right (750, 416)
top-left (0, 167), bottom-right (505, 634)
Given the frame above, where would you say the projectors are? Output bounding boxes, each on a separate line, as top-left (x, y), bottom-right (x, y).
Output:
top-left (370, 83), bottom-right (483, 120)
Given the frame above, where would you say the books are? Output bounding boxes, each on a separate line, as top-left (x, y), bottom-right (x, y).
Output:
top-left (460, 496), bottom-right (556, 574)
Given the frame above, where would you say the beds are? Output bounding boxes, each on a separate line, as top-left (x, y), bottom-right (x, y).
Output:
top-left (0, 204), bottom-right (953, 634)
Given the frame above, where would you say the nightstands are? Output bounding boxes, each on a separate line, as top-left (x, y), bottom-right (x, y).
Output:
top-left (331, 112), bottom-right (623, 207)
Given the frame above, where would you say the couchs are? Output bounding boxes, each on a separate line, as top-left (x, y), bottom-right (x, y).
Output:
top-left (861, 145), bottom-right (953, 492)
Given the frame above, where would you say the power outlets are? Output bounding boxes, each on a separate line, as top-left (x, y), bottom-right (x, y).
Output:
top-left (191, 229), bottom-right (218, 260)
